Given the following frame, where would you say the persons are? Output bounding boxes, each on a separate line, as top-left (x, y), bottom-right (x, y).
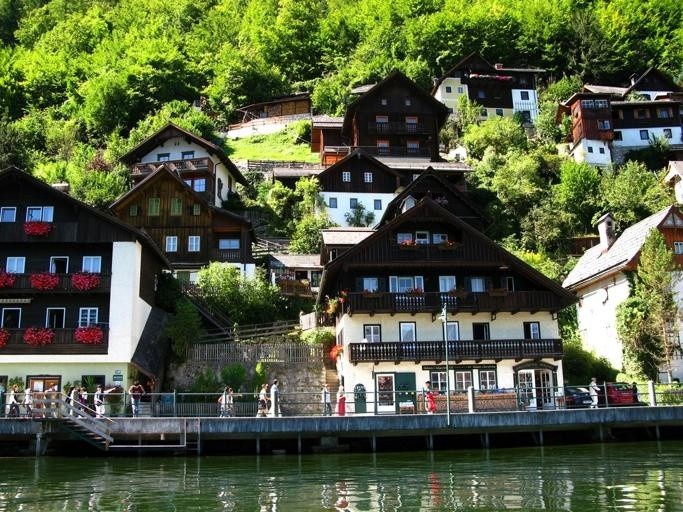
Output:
top-left (335, 382), bottom-right (347, 416)
top-left (4, 384), bottom-right (89, 420)
top-left (225, 388), bottom-right (236, 417)
top-left (267, 378), bottom-right (283, 418)
top-left (216, 387), bottom-right (230, 417)
top-left (320, 382), bottom-right (332, 416)
top-left (127, 381), bottom-right (145, 418)
top-left (424, 380), bottom-right (435, 415)
top-left (254, 384), bottom-right (269, 417)
top-left (588, 378), bottom-right (602, 409)
top-left (93, 384), bottom-right (116, 418)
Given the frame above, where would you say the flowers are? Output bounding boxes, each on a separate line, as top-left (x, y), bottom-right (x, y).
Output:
top-left (0, 221), bottom-right (102, 346)
top-left (335, 238), bottom-right (466, 300)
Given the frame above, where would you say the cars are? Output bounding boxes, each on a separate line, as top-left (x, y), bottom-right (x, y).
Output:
top-left (565, 381), bottom-right (634, 406)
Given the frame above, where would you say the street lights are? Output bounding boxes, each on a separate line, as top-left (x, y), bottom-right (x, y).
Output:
top-left (439, 302), bottom-right (451, 426)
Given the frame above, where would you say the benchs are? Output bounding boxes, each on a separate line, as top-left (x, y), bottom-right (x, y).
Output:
top-left (398, 401), bottom-right (415, 414)
